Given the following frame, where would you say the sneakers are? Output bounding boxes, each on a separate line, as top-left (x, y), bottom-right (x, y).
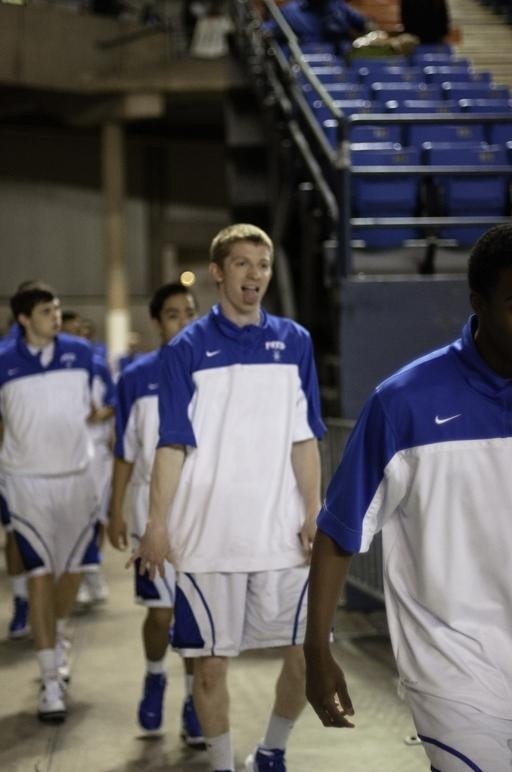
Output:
top-left (244, 743), bottom-right (290, 772)
top-left (34, 661), bottom-right (73, 722)
top-left (44, 635), bottom-right (76, 679)
top-left (6, 594), bottom-right (35, 640)
top-left (179, 694), bottom-right (206, 748)
top-left (137, 671), bottom-right (168, 732)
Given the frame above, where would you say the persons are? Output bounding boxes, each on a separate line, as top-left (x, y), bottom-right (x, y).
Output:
top-left (0, 274), bottom-right (119, 725)
top-left (104, 281), bottom-right (210, 748)
top-left (125, 223), bottom-right (328, 772)
top-left (303, 221), bottom-right (510, 770)
top-left (0, 310), bottom-right (112, 637)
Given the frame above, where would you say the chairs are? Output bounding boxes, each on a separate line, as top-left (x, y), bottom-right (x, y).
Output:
top-left (275, 40), bottom-right (509, 274)
